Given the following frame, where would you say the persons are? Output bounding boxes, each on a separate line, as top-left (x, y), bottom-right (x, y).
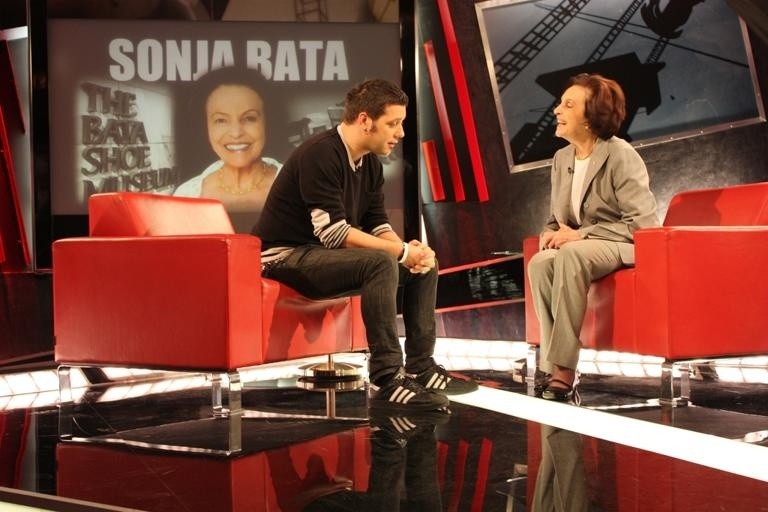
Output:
top-left (292, 409), bottom-right (464, 511)
top-left (251, 77), bottom-right (475, 411)
top-left (525, 72), bottom-right (662, 401)
top-left (174, 68), bottom-right (298, 231)
top-left (524, 423), bottom-right (595, 511)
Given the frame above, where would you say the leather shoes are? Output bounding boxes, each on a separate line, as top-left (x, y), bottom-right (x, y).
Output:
top-left (534, 373), bottom-right (552, 394)
top-left (540, 375), bottom-right (581, 402)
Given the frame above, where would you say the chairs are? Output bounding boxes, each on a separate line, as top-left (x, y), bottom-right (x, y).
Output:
top-left (50, 192), bottom-right (373, 416)
top-left (522, 182), bottom-right (768, 406)
top-left (524, 379), bottom-right (768, 511)
top-left (56, 375), bottom-right (375, 512)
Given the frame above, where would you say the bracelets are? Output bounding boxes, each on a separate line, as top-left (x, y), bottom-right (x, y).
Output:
top-left (397, 244), bottom-right (411, 263)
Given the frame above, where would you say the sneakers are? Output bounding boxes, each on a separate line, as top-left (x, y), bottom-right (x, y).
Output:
top-left (367, 366), bottom-right (450, 412)
top-left (366, 406), bottom-right (452, 450)
top-left (402, 357), bottom-right (479, 395)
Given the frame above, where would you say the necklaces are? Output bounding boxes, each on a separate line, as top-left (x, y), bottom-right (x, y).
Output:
top-left (575, 148), bottom-right (594, 160)
top-left (215, 163), bottom-right (271, 197)
